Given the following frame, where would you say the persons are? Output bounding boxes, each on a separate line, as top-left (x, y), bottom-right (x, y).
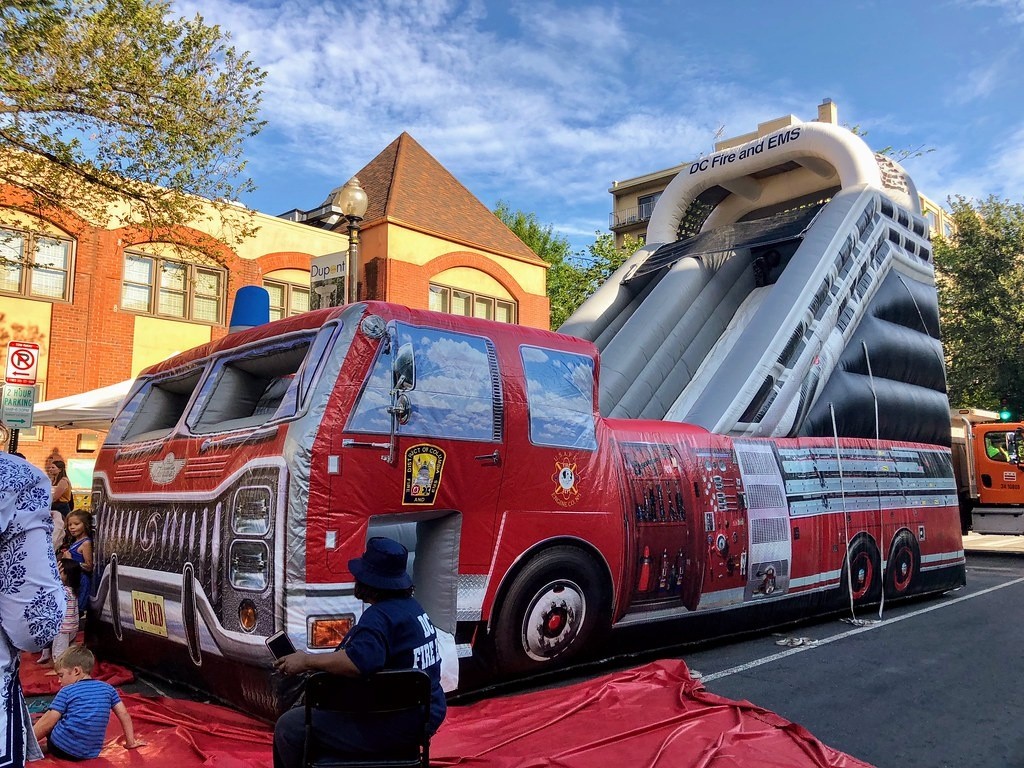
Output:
top-left (987, 436), bottom-right (1008, 461)
top-left (0, 450), bottom-right (146, 768)
top-left (272, 536), bottom-right (446, 768)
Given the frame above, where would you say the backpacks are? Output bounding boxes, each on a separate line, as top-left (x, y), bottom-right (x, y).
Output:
top-left (60, 479), bottom-right (74, 511)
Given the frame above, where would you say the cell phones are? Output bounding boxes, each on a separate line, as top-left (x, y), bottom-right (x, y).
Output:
top-left (265, 630), bottom-right (298, 675)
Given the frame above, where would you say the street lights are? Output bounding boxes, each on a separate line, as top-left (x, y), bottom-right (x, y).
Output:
top-left (340, 174), bottom-right (370, 304)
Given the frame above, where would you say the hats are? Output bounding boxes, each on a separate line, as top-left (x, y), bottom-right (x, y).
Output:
top-left (348, 537), bottom-right (412, 590)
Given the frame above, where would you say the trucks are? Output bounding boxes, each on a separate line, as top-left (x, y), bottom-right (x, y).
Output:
top-left (950, 406), bottom-right (1024, 537)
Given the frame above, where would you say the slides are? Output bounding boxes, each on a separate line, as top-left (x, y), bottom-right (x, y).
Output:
top-left (557, 190), bottom-right (858, 431)
